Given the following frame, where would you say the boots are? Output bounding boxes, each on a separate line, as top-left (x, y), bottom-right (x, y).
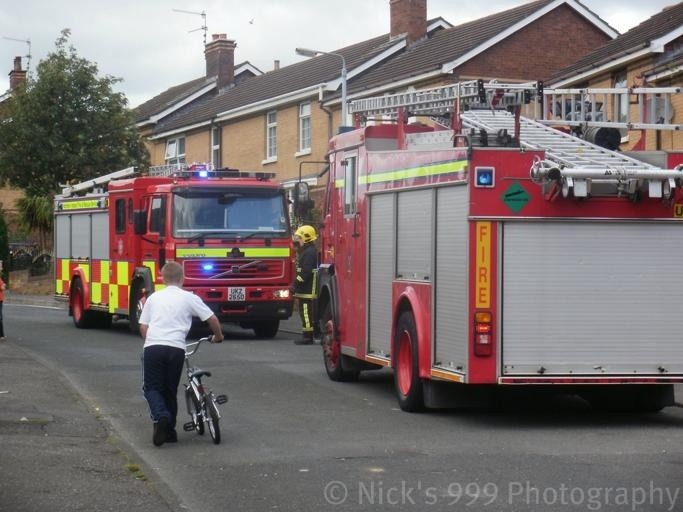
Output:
top-left (293, 327), bottom-right (314, 345)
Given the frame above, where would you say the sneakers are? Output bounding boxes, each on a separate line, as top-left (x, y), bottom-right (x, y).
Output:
top-left (150, 413), bottom-right (170, 447)
top-left (167, 427), bottom-right (178, 444)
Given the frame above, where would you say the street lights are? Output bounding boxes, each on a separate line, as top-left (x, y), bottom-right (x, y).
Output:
top-left (293, 45), bottom-right (349, 135)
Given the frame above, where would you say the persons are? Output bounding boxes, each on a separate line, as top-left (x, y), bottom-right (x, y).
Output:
top-left (292, 225), bottom-right (320, 345)
top-left (138, 262), bottom-right (224, 446)
top-left (0, 278), bottom-right (7, 339)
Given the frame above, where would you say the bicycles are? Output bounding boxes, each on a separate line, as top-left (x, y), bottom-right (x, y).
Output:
top-left (182, 333), bottom-right (228, 445)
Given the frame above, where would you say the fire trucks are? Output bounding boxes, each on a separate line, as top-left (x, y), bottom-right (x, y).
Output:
top-left (53, 160), bottom-right (296, 340)
top-left (294, 69), bottom-right (683, 417)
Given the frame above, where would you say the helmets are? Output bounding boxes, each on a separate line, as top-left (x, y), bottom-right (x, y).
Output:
top-left (293, 225), bottom-right (318, 246)
